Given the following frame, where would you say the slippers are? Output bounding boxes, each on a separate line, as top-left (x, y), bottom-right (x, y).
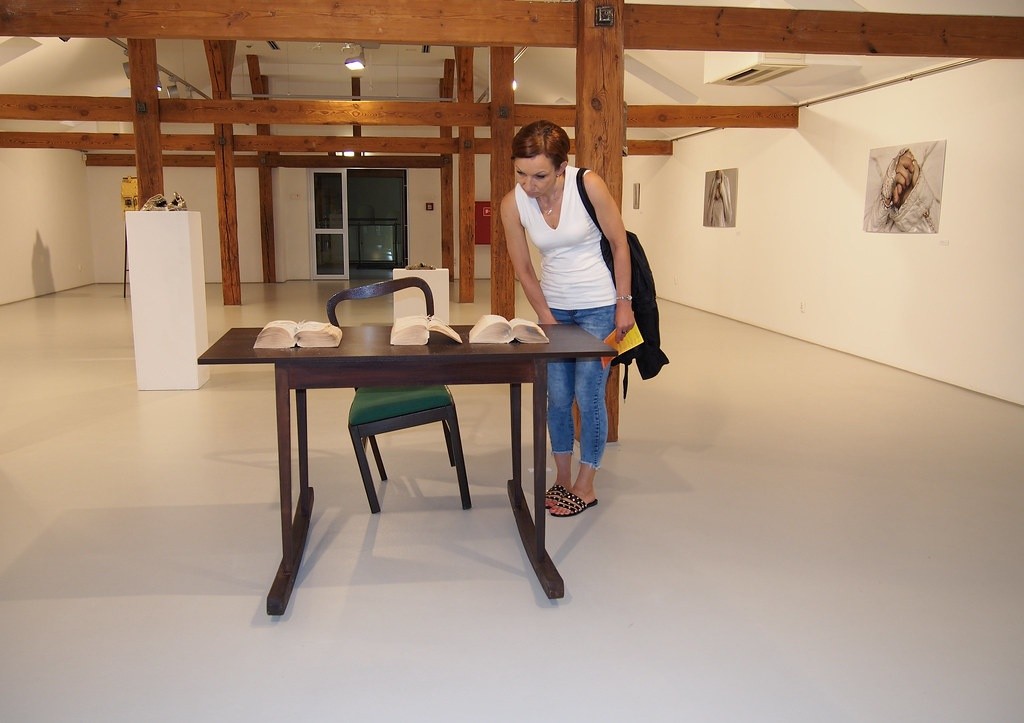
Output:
top-left (545, 484), bottom-right (571, 509)
top-left (551, 491), bottom-right (598, 518)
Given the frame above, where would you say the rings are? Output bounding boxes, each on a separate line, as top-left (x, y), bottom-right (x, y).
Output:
top-left (622, 329), bottom-right (627, 334)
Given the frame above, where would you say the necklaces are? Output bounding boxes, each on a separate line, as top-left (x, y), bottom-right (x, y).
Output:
top-left (537, 197), bottom-right (555, 215)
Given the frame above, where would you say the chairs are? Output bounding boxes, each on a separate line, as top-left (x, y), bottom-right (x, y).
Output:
top-left (325, 276), bottom-right (472, 514)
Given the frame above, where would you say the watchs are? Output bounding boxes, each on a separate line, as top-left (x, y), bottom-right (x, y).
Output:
top-left (616, 295), bottom-right (633, 301)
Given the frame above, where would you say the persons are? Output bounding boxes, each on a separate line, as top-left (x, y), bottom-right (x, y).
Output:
top-left (499, 121), bottom-right (634, 518)
top-left (864, 147), bottom-right (941, 233)
top-left (707, 170), bottom-right (733, 228)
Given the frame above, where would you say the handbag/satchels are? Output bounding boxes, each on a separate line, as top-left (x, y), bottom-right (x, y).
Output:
top-left (599, 229), bottom-right (671, 401)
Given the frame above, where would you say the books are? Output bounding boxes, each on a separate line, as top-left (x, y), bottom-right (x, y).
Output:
top-left (468, 315), bottom-right (550, 344)
top-left (390, 315), bottom-right (462, 346)
top-left (253, 320), bottom-right (342, 349)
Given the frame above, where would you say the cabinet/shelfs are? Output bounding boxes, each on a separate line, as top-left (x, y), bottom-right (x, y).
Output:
top-left (123, 209), bottom-right (209, 390)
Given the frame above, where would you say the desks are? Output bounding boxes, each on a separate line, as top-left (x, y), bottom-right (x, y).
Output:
top-left (198, 324), bottom-right (618, 615)
top-left (392, 268), bottom-right (451, 325)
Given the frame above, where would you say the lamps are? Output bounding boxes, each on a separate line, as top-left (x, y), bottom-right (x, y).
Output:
top-left (343, 50), bottom-right (366, 70)
